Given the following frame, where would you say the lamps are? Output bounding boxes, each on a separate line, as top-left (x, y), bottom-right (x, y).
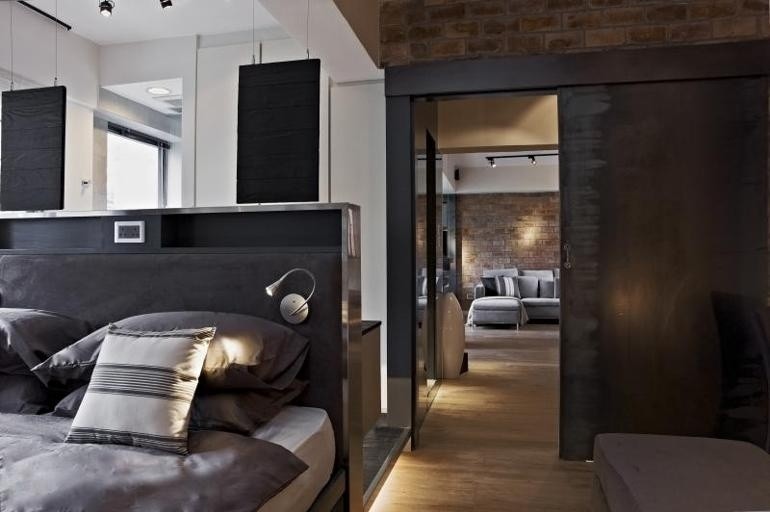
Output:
top-left (264, 267), bottom-right (317, 326)
top-left (159, 0), bottom-right (173, 12)
top-left (98, 0), bottom-right (115, 18)
top-left (488, 154), bottom-right (540, 169)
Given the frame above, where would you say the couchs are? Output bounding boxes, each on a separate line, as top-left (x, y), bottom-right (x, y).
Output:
top-left (463, 269), bottom-right (527, 331)
top-left (520, 268), bottom-right (560, 321)
top-left (415, 267), bottom-right (451, 329)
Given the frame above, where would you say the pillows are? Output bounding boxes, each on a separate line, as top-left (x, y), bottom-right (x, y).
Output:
top-left (29, 309), bottom-right (312, 394)
top-left (0, 371), bottom-right (73, 415)
top-left (55, 379), bottom-right (312, 439)
top-left (64, 319), bottom-right (217, 459)
top-left (0, 305), bottom-right (96, 387)
top-left (481, 274), bottom-right (560, 299)
top-left (421, 276), bottom-right (440, 296)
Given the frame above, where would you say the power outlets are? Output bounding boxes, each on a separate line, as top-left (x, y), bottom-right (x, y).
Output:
top-left (114, 220), bottom-right (147, 245)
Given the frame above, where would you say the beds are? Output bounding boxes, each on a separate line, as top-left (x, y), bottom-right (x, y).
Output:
top-left (0, 202), bottom-right (362, 511)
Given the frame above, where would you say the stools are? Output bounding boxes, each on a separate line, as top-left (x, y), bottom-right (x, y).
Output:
top-left (596, 433), bottom-right (770, 511)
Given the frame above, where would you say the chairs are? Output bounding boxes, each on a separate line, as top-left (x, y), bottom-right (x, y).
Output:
top-left (707, 290), bottom-right (768, 441)
